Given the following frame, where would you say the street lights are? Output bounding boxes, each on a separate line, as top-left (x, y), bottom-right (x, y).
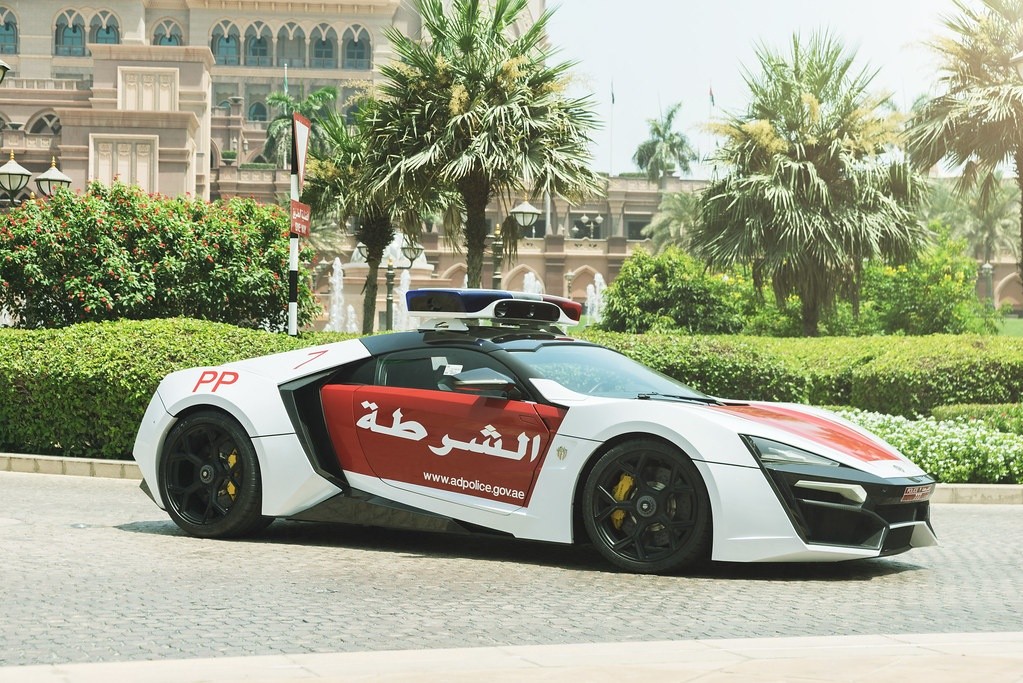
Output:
top-left (981, 260), bottom-right (993, 300)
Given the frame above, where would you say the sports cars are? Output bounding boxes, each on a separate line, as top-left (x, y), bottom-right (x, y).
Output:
top-left (132, 287), bottom-right (939, 576)
